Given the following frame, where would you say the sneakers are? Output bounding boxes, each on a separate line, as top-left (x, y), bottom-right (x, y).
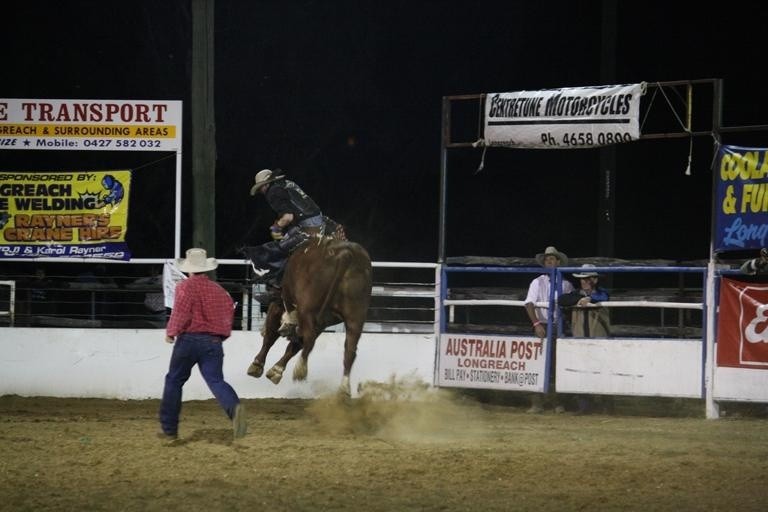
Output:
top-left (233, 404), bottom-right (248, 438)
top-left (157, 432), bottom-right (179, 444)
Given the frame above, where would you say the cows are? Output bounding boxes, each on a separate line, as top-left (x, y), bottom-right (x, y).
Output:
top-left (246, 237), bottom-right (374, 407)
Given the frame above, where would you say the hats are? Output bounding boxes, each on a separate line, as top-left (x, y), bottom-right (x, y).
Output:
top-left (534, 246), bottom-right (569, 266)
top-left (175, 247), bottom-right (218, 274)
top-left (250, 168), bottom-right (287, 196)
top-left (573, 263), bottom-right (601, 278)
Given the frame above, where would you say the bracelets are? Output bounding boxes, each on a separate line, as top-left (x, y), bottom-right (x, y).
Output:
top-left (532, 319), bottom-right (542, 327)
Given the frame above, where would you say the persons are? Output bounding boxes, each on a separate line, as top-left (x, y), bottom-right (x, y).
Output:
top-left (558, 263), bottom-right (615, 425)
top-left (250, 168), bottom-right (326, 304)
top-left (524, 244), bottom-right (576, 414)
top-left (154, 247), bottom-right (248, 446)
